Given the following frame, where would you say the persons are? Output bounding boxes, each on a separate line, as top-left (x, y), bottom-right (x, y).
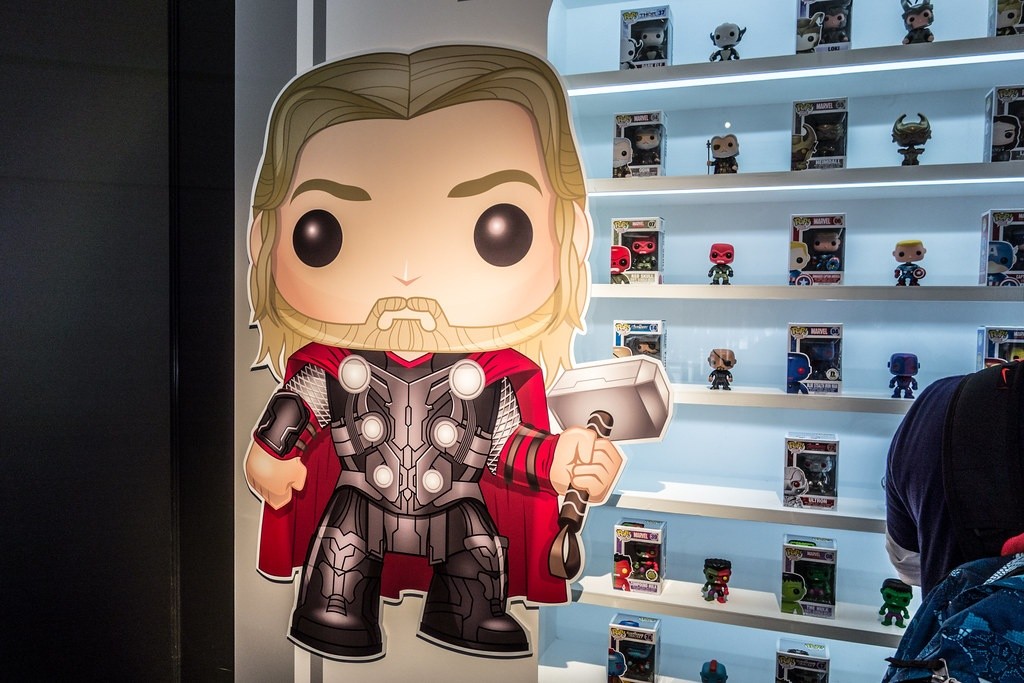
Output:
top-left (807, 563), bottom-right (832, 602)
top-left (708, 21), bottom-right (747, 62)
top-left (708, 243), bottom-right (734, 284)
top-left (701, 559), bottom-right (732, 603)
top-left (707, 349), bottom-right (737, 390)
top-left (816, 113), bottom-right (845, 155)
top-left (808, 229), bottom-right (841, 271)
top-left (626, 642), bottom-right (653, 680)
top-left (804, 453), bottom-right (832, 495)
top-left (879, 578), bottom-right (913, 628)
top-left (885, 358), bottom-right (1024, 683)
top-left (631, 124), bottom-right (661, 165)
top-left (900, 0), bottom-right (936, 46)
top-left (631, 234), bottom-right (658, 270)
top-left (628, 335), bottom-right (660, 360)
top-left (810, 340), bottom-right (840, 380)
top-left (888, 352), bottom-right (921, 398)
top-left (632, 541), bottom-right (658, 581)
top-left (707, 135), bottom-right (739, 174)
top-left (700, 661), bottom-right (728, 683)
top-left (635, 20), bottom-right (667, 61)
top-left (892, 112), bottom-right (931, 166)
top-left (892, 241), bottom-right (927, 286)
top-left (819, 0), bottom-right (851, 43)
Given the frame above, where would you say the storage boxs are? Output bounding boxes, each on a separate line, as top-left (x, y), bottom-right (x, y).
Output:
top-left (775, 638), bottom-right (831, 683)
top-left (982, 85), bottom-right (1024, 162)
top-left (613, 110), bottom-right (668, 178)
top-left (606, 613), bottom-right (663, 683)
top-left (610, 217), bottom-right (665, 285)
top-left (795, 0), bottom-right (854, 54)
top-left (976, 325), bottom-right (1024, 372)
top-left (987, 0), bottom-right (1024, 37)
top-left (783, 432), bottom-right (840, 511)
top-left (790, 97), bottom-right (849, 171)
top-left (786, 322), bottom-right (844, 396)
top-left (978, 208), bottom-right (1024, 287)
top-left (779, 533), bottom-right (839, 619)
top-left (619, 5), bottom-right (674, 70)
top-left (613, 319), bottom-right (667, 371)
top-left (789, 212), bottom-right (846, 286)
top-left (611, 517), bottom-right (669, 595)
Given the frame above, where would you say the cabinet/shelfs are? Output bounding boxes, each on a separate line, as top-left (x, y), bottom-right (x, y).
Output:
top-left (538, 0), bottom-right (1024, 683)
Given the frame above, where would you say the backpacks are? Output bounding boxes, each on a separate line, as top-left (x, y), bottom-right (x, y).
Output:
top-left (881, 557), bottom-right (1023, 683)
